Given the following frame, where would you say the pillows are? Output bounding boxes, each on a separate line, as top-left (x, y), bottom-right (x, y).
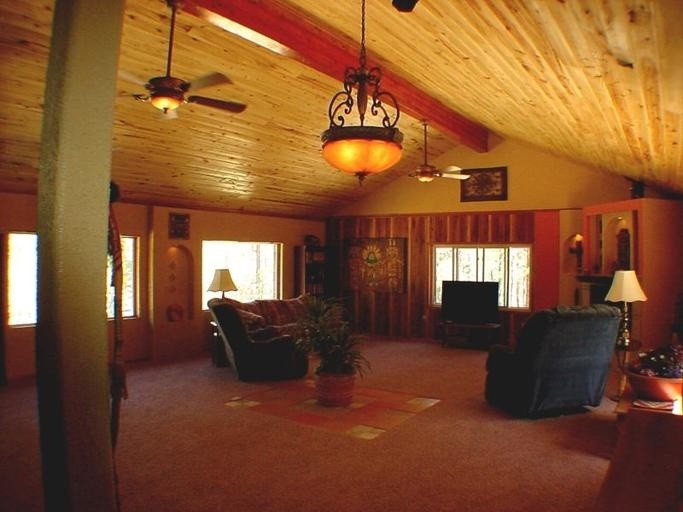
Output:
top-left (235, 309), bottom-right (265, 327)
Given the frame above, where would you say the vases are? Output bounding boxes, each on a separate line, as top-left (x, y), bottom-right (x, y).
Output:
top-left (625, 370), bottom-right (681, 398)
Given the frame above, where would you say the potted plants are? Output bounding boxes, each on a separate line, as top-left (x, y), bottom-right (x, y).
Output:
top-left (296, 289), bottom-right (364, 410)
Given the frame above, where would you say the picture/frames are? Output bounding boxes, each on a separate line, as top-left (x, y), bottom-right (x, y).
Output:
top-left (456, 166), bottom-right (509, 202)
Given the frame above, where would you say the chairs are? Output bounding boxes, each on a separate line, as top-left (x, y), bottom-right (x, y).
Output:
top-left (207, 295), bottom-right (314, 379)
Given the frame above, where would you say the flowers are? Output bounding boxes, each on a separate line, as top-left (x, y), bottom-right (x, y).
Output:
top-left (629, 327), bottom-right (682, 377)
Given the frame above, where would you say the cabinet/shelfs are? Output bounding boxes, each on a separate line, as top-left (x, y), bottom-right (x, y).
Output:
top-left (438, 321), bottom-right (498, 351)
top-left (601, 394), bottom-right (682, 512)
top-left (617, 338), bottom-right (635, 401)
top-left (295, 244), bottom-right (327, 301)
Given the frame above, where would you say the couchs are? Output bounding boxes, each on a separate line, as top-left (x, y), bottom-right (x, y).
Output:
top-left (485, 305), bottom-right (622, 416)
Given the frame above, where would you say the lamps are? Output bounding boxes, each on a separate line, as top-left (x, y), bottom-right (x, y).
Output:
top-left (603, 270), bottom-right (647, 397)
top-left (318, 0), bottom-right (401, 180)
top-left (146, 93), bottom-right (184, 117)
top-left (207, 268), bottom-right (237, 298)
top-left (413, 174), bottom-right (434, 182)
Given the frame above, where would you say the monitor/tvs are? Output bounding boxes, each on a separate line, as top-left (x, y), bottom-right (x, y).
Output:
top-left (442, 280), bottom-right (498, 324)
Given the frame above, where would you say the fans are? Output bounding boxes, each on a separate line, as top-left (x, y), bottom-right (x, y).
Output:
top-left (112, 0), bottom-right (246, 118)
top-left (403, 119), bottom-right (471, 183)
